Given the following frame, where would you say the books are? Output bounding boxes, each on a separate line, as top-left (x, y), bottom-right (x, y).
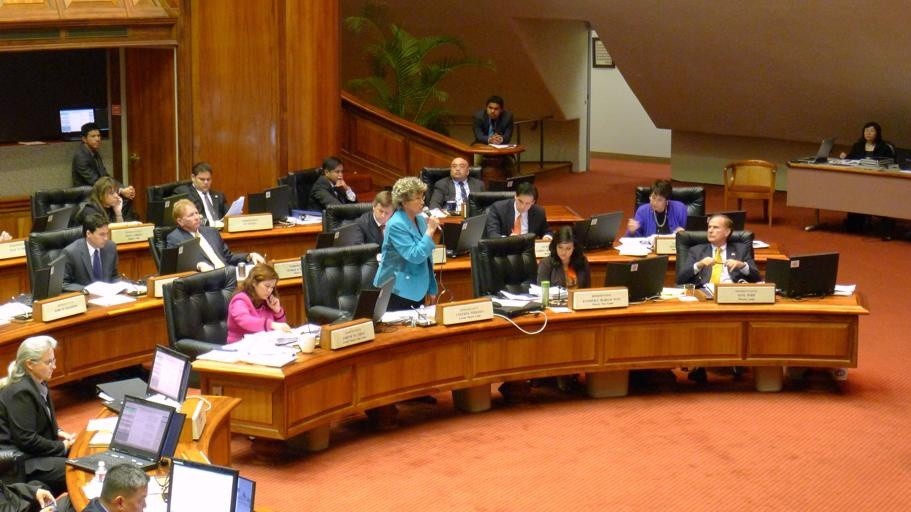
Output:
top-left (84, 281), bottom-right (147, 297)
top-left (834, 155), bottom-right (901, 171)
top-left (196, 324), bottom-right (320, 366)
top-left (219, 197), bottom-right (245, 222)
top-left (616, 232), bottom-right (654, 256)
top-left (490, 142), bottom-right (517, 149)
top-left (96, 378), bottom-right (150, 407)
top-left (288, 210), bottom-right (324, 225)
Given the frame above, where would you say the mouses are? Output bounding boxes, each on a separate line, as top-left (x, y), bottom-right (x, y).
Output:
top-left (493, 302), bottom-right (501, 307)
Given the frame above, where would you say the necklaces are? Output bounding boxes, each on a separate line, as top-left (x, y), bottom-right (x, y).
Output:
top-left (651, 200), bottom-right (671, 229)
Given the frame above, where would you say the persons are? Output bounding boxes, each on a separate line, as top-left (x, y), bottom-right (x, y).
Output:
top-left (174, 160), bottom-right (244, 227)
top-left (0, 231), bottom-right (13, 243)
top-left (356, 190), bottom-right (398, 252)
top-left (72, 125), bottom-right (136, 219)
top-left (67, 175), bottom-right (125, 227)
top-left (1, 335), bottom-right (74, 491)
top-left (487, 181), bottom-right (555, 243)
top-left (429, 157), bottom-right (486, 213)
top-left (537, 223), bottom-right (593, 290)
top-left (839, 121), bottom-right (896, 237)
top-left (1, 476), bottom-right (59, 511)
top-left (167, 198), bottom-right (268, 272)
top-left (226, 263), bottom-right (291, 345)
top-left (373, 176), bottom-right (441, 406)
top-left (82, 463), bottom-right (151, 511)
top-left (676, 215), bottom-right (762, 384)
top-left (471, 95), bottom-right (514, 143)
top-left (620, 174), bottom-right (687, 237)
top-left (59, 215), bottom-right (120, 293)
top-left (308, 157), bottom-right (359, 212)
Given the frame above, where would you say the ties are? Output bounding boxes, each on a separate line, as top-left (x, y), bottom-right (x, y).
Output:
top-left (203, 192), bottom-right (219, 221)
top-left (380, 224), bottom-right (385, 235)
top-left (195, 232), bottom-right (225, 269)
top-left (93, 250), bottom-right (101, 280)
top-left (458, 181), bottom-right (467, 200)
top-left (514, 212), bottom-right (521, 235)
top-left (710, 248), bottom-right (722, 285)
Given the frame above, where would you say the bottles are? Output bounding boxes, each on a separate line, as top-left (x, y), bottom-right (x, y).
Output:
top-left (95, 460), bottom-right (108, 488)
top-left (540, 280), bottom-right (549, 307)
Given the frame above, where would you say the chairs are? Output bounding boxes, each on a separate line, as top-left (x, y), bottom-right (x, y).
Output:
top-left (723, 159), bottom-right (777, 228)
top-left (1, 165), bottom-right (792, 390)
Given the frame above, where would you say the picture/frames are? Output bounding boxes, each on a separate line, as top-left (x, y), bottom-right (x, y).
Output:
top-left (591, 37), bottom-right (615, 68)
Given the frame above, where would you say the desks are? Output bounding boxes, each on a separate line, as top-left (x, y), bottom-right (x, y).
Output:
top-left (784, 159), bottom-right (911, 233)
top-left (434, 114), bottom-right (554, 172)
top-left (2, 238), bottom-right (790, 390)
top-left (190, 287), bottom-right (867, 454)
top-left (64, 396), bottom-right (266, 512)
top-left (1, 203), bottom-right (584, 302)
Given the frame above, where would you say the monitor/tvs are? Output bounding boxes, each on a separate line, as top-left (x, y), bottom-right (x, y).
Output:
top-left (59, 108), bottom-right (96, 142)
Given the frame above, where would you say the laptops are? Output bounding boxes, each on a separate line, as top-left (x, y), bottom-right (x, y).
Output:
top-left (765, 253), bottom-right (839, 299)
top-left (167, 457), bottom-right (239, 512)
top-left (494, 299), bottom-right (546, 316)
top-left (65, 394), bottom-right (176, 477)
top-left (353, 278), bottom-right (394, 324)
top-left (575, 211), bottom-right (624, 250)
top-left (686, 211), bottom-right (746, 231)
top-left (488, 174), bottom-right (535, 191)
top-left (797, 137), bottom-right (834, 163)
top-left (605, 256), bottom-right (669, 301)
top-left (438, 214), bottom-right (488, 258)
top-left (101, 343), bottom-right (192, 416)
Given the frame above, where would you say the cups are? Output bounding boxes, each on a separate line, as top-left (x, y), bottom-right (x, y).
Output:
top-left (298, 333), bottom-right (316, 353)
top-left (684, 284), bottom-right (697, 297)
top-left (446, 200), bottom-right (458, 213)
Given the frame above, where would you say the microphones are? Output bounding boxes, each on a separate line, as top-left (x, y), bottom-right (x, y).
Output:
top-left (423, 206), bottom-right (442, 230)
